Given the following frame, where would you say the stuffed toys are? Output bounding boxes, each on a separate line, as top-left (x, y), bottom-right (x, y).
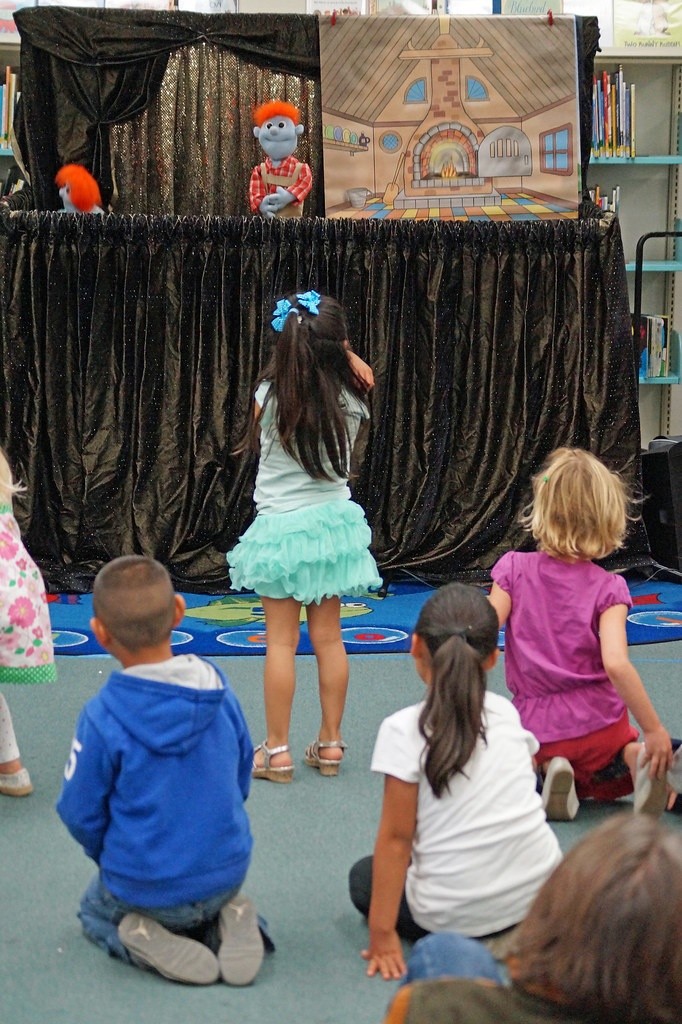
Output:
top-left (54, 164), bottom-right (105, 214)
top-left (249, 101), bottom-right (312, 217)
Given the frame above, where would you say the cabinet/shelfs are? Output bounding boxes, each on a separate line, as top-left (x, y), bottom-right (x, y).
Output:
top-left (588, 56), bottom-right (682, 436)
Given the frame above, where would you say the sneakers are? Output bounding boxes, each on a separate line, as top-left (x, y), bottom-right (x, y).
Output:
top-left (541, 755), bottom-right (581, 822)
top-left (633, 740), bottom-right (670, 821)
top-left (217, 894), bottom-right (264, 986)
top-left (0, 765), bottom-right (34, 800)
top-left (117, 910), bottom-right (220, 985)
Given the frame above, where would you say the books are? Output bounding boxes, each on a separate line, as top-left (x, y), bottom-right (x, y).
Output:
top-left (0, 66), bottom-right (22, 150)
top-left (589, 185), bottom-right (620, 212)
top-left (630, 313), bottom-right (672, 379)
top-left (590, 64), bottom-right (636, 159)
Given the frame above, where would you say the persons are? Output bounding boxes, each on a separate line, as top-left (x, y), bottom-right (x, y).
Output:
top-left (348, 450), bottom-right (681, 1024)
top-left (56, 554), bottom-right (276, 987)
top-left (0, 435), bottom-right (56, 794)
top-left (227, 292), bottom-right (384, 782)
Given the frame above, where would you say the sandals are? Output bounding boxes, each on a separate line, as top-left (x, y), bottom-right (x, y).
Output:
top-left (304, 736), bottom-right (348, 777)
top-left (251, 739), bottom-right (295, 784)
